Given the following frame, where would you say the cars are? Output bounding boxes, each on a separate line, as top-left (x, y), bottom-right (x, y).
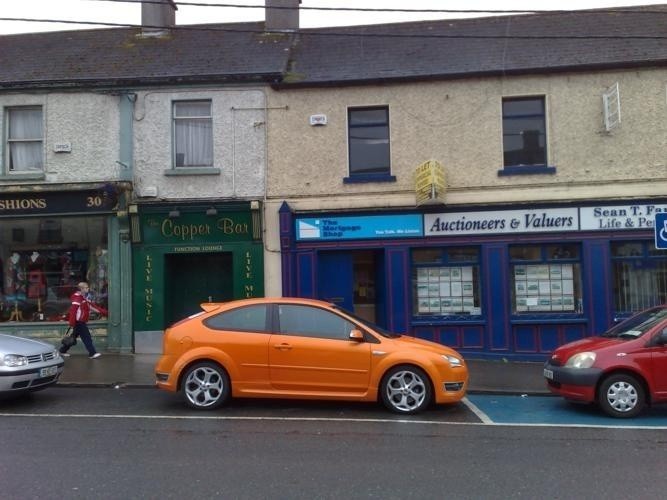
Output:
top-left (542, 301), bottom-right (666, 418)
top-left (0, 333), bottom-right (65, 402)
top-left (154, 297), bottom-right (468, 415)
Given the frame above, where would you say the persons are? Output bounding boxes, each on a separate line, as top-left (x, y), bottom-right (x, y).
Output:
top-left (2, 252), bottom-right (24, 295)
top-left (59, 282), bottom-right (108, 359)
top-left (25, 251), bottom-right (48, 299)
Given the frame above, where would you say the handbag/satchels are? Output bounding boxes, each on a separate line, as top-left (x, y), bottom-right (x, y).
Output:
top-left (62, 336), bottom-right (76, 346)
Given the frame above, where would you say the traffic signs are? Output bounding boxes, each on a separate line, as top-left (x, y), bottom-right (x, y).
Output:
top-left (654, 212), bottom-right (666, 249)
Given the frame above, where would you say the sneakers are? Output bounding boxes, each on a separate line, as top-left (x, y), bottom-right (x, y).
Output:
top-left (60, 352), bottom-right (71, 357)
top-left (89, 352), bottom-right (101, 359)
